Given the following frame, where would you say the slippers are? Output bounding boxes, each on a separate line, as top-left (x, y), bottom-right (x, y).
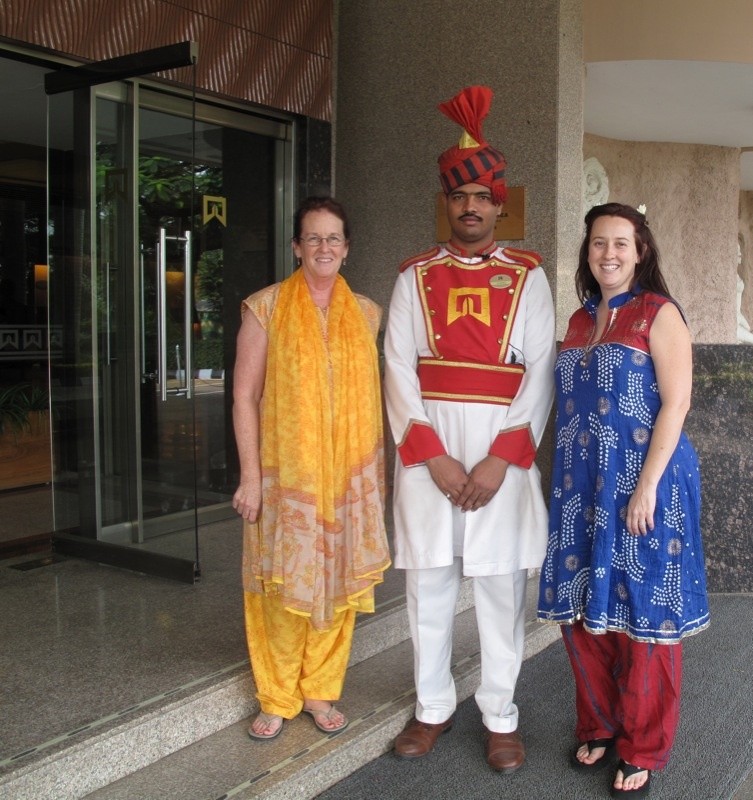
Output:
top-left (248, 712), bottom-right (283, 740)
top-left (570, 737), bottom-right (615, 771)
top-left (610, 757), bottom-right (652, 800)
top-left (300, 702), bottom-right (349, 732)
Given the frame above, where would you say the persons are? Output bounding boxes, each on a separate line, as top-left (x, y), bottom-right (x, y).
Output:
top-left (232, 195), bottom-right (392, 741)
top-left (536, 202), bottom-right (712, 800)
top-left (383, 144), bottom-right (557, 777)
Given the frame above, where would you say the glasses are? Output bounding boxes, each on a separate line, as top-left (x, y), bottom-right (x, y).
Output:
top-left (301, 234), bottom-right (345, 247)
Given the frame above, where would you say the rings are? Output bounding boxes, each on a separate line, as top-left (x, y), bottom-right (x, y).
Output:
top-left (446, 493), bottom-right (451, 498)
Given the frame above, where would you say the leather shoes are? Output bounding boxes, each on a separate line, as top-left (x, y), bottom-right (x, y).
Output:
top-left (394, 717), bottom-right (452, 758)
top-left (485, 727), bottom-right (525, 773)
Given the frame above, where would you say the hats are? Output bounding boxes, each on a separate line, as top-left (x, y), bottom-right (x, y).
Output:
top-left (438, 86), bottom-right (509, 204)
